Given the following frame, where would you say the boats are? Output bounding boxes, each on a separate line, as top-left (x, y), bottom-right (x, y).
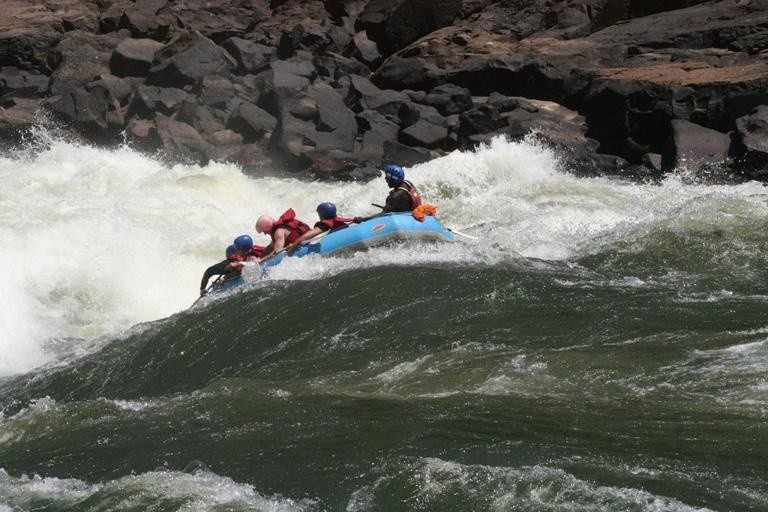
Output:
top-left (212, 210), bottom-right (454, 300)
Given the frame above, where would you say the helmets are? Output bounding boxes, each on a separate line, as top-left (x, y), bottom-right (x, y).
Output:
top-left (316, 202), bottom-right (336, 217)
top-left (384, 164), bottom-right (405, 180)
top-left (255, 215), bottom-right (275, 234)
top-left (225, 235), bottom-right (252, 256)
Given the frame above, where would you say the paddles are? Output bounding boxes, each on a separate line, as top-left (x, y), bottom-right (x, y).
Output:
top-left (372, 203), bottom-right (480, 240)
top-left (231, 246), bottom-right (290, 267)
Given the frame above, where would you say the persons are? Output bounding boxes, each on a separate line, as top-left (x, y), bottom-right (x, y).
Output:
top-left (200, 165), bottom-right (422, 296)
